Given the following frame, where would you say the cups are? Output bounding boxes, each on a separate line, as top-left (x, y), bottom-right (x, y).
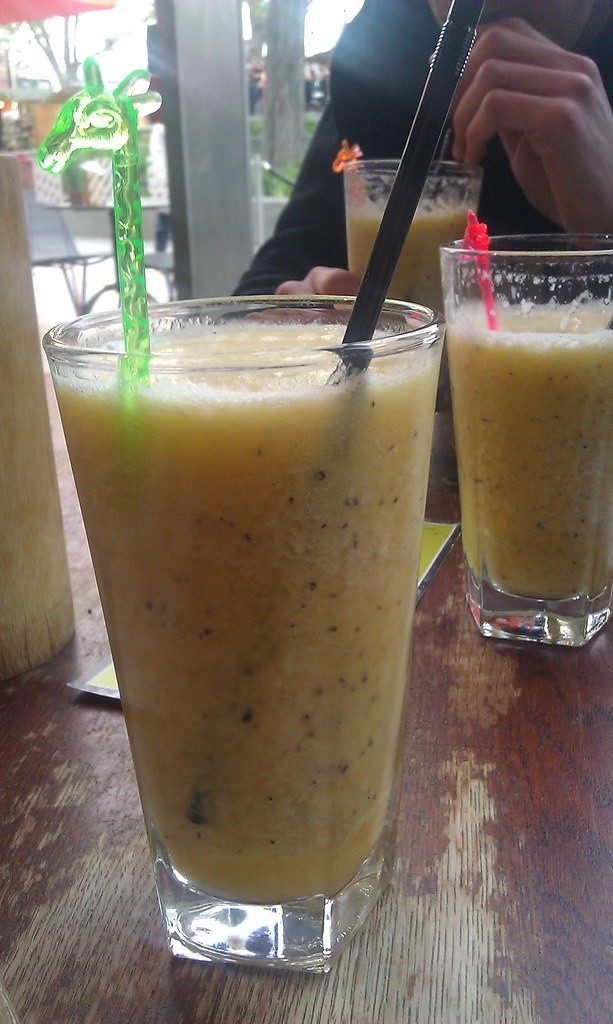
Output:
top-left (342, 158), bottom-right (483, 314)
top-left (42, 295), bottom-right (447, 972)
top-left (439, 234), bottom-right (613, 647)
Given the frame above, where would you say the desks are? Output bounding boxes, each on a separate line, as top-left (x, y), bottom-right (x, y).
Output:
top-left (42, 200), bottom-right (171, 307)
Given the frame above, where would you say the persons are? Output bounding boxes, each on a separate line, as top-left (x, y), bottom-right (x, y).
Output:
top-left (245, 66), bottom-right (263, 115)
top-left (146, 72), bottom-right (176, 253)
top-left (306, 67), bottom-right (331, 109)
top-left (219, 0), bottom-right (613, 325)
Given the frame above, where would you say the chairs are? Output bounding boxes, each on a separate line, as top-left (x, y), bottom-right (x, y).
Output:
top-left (25, 204), bottom-right (174, 319)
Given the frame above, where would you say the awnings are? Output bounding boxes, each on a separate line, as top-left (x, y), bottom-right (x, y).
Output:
top-left (0, 0), bottom-right (115, 25)
